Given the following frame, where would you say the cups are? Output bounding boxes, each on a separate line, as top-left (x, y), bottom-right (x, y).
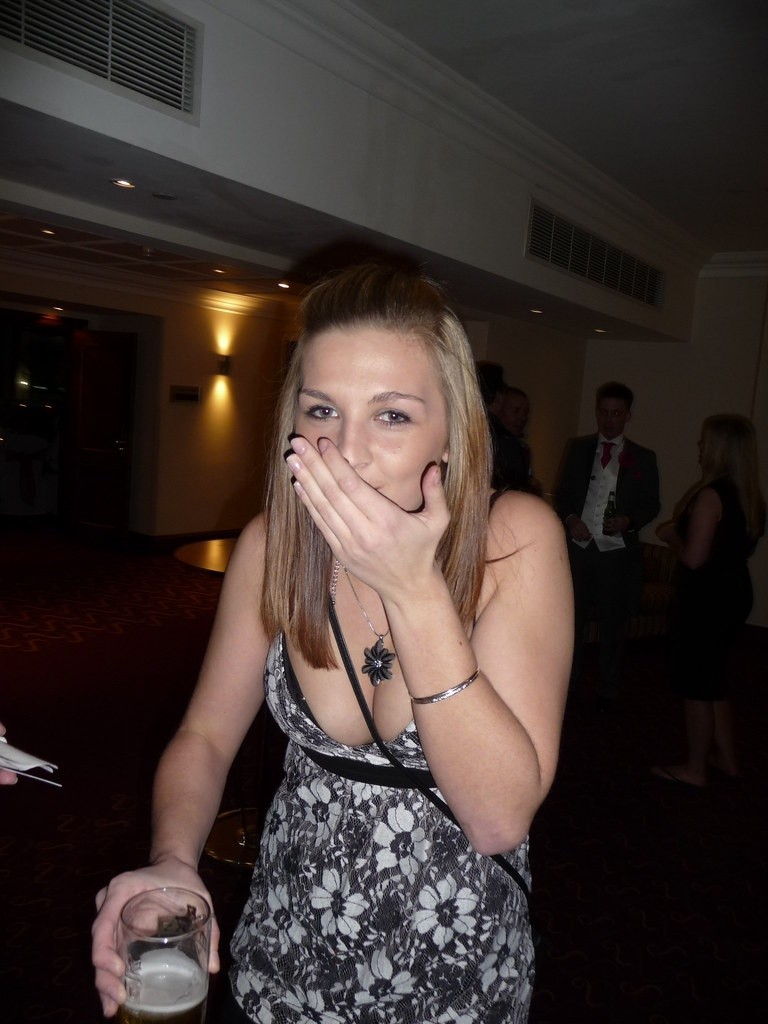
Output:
top-left (119, 887), bottom-right (212, 1024)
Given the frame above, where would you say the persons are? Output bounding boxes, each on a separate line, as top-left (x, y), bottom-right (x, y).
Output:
top-left (92, 264), bottom-right (575, 1023)
top-left (475, 360), bottom-right (543, 498)
top-left (558, 381), bottom-right (661, 724)
top-left (649, 413), bottom-right (768, 796)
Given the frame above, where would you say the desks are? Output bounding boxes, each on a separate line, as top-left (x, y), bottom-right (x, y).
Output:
top-left (201, 805), bottom-right (274, 997)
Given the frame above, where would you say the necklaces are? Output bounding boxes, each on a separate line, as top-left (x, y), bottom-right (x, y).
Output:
top-left (331, 559), bottom-right (341, 605)
top-left (342, 564), bottom-right (396, 686)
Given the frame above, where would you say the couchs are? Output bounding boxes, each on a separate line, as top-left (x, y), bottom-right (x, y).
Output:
top-left (576, 541), bottom-right (679, 659)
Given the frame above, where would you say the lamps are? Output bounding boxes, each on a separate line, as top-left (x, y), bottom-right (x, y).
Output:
top-left (215, 355), bottom-right (233, 376)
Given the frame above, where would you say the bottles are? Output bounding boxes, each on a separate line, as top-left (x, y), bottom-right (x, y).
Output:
top-left (602, 492), bottom-right (617, 536)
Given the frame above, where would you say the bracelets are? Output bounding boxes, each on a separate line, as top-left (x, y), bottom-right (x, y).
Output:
top-left (408, 666), bottom-right (480, 704)
